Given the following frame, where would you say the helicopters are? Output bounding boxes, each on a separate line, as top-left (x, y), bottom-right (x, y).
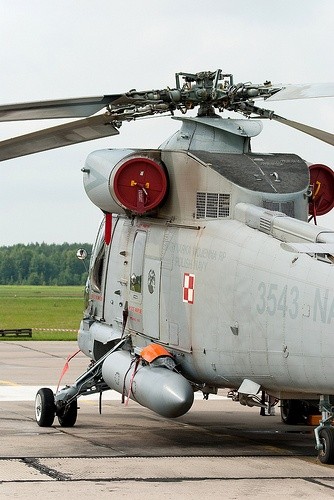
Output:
top-left (0, 69), bottom-right (334, 468)
